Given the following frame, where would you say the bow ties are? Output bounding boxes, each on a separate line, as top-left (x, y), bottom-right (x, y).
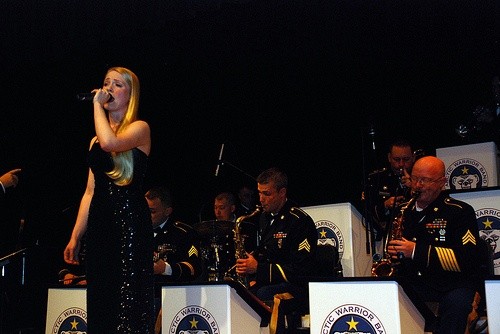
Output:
top-left (410, 210), bottom-right (425, 219)
top-left (268, 214), bottom-right (277, 222)
top-left (152, 226), bottom-right (161, 234)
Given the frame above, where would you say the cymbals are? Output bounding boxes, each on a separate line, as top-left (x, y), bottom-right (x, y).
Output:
top-left (193, 220), bottom-right (235, 236)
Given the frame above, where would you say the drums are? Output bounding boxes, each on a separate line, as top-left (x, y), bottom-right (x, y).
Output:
top-left (201, 234), bottom-right (234, 277)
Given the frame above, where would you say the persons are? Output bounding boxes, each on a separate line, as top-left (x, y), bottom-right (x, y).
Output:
top-left (367, 135), bottom-right (422, 235)
top-left (236, 184), bottom-right (257, 218)
top-left (213, 192), bottom-right (239, 225)
top-left (382, 155), bottom-right (480, 334)
top-left (453, 76), bottom-right (500, 155)
top-left (236, 168), bottom-right (318, 334)
top-left (143, 187), bottom-right (201, 285)
top-left (63, 67), bottom-right (159, 334)
top-left (58, 233), bottom-right (87, 287)
top-left (0, 168), bottom-right (22, 195)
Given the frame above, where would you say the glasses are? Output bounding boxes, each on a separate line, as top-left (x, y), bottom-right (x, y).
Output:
top-left (390, 155), bottom-right (413, 164)
top-left (409, 176), bottom-right (442, 186)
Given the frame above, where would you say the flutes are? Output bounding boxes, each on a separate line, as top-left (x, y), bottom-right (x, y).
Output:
top-left (385, 170), bottom-right (403, 215)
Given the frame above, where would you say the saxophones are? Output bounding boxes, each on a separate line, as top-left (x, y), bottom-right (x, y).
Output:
top-left (371, 190), bottom-right (422, 278)
top-left (224, 206), bottom-right (264, 283)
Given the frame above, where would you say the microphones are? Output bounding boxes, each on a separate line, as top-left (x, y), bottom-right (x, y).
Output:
top-left (78, 93), bottom-right (112, 103)
top-left (215, 144), bottom-right (225, 176)
top-left (370, 129), bottom-right (376, 150)
